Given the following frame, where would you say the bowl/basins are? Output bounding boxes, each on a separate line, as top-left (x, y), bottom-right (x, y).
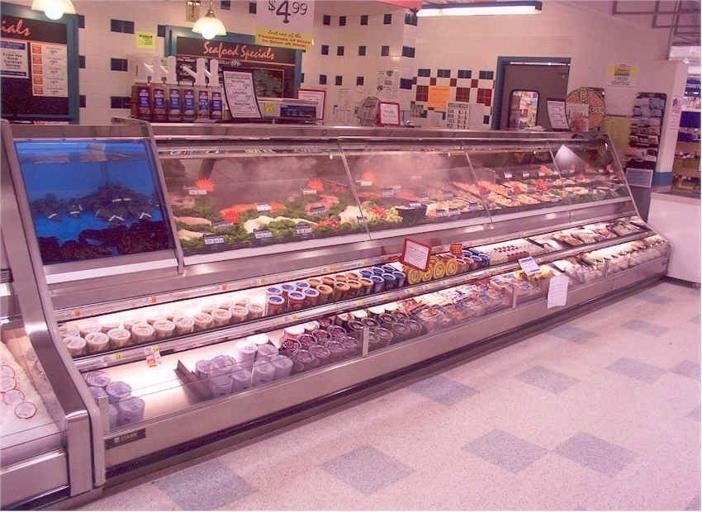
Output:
top-left (58, 297), bottom-right (263, 358)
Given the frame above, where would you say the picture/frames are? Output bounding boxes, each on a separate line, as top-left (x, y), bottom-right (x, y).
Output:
top-left (294, 87), bottom-right (327, 121)
top-left (377, 101), bottom-right (401, 127)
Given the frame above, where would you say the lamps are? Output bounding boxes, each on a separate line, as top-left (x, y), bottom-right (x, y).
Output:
top-left (191, 0), bottom-right (230, 42)
top-left (31, 0), bottom-right (79, 21)
top-left (414, 0), bottom-right (542, 18)
